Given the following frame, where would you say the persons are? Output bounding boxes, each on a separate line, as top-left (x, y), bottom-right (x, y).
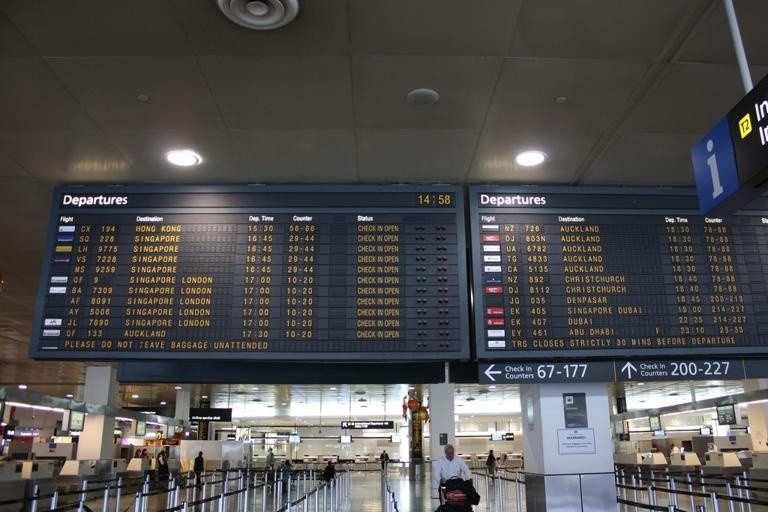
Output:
top-left (134, 448), bottom-right (168, 479)
top-left (432, 445), bottom-right (472, 501)
top-left (380, 450), bottom-right (389, 470)
top-left (266, 447), bottom-right (274, 469)
top-left (194, 451), bottom-right (204, 487)
top-left (324, 461), bottom-right (336, 487)
top-left (486, 450), bottom-right (496, 482)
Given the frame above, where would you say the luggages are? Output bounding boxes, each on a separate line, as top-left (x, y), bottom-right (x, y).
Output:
top-left (448, 490), bottom-right (471, 507)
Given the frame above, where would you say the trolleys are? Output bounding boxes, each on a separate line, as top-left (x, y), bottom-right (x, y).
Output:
top-left (438, 480), bottom-right (473, 512)
